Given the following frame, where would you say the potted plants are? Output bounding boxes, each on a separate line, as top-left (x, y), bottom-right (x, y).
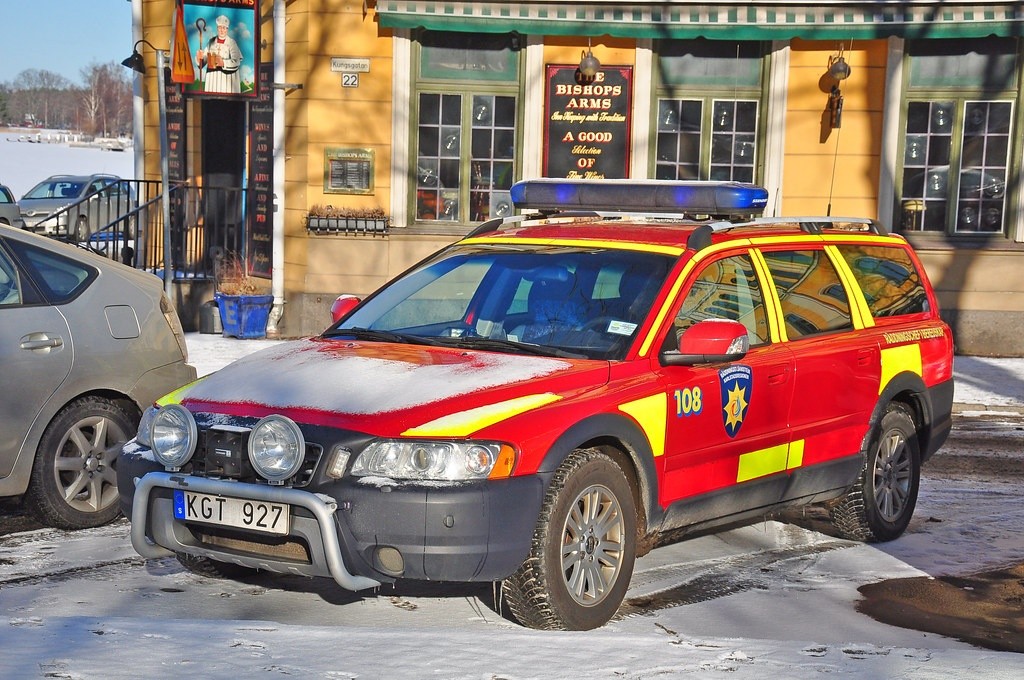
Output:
top-left (307, 204), bottom-right (389, 231)
top-left (215, 248), bottom-right (274, 340)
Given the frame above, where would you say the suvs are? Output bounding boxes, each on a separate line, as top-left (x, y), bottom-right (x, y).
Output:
top-left (122, 178), bottom-right (960, 637)
top-left (19, 176), bottom-right (138, 242)
top-left (0, 223), bottom-right (214, 526)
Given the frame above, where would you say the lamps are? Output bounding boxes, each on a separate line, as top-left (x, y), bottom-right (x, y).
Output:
top-left (579, 37), bottom-right (600, 77)
top-left (120, 40), bottom-right (170, 74)
top-left (829, 42), bottom-right (851, 80)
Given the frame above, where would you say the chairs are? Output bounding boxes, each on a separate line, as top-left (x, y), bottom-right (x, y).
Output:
top-left (507, 271), bottom-right (601, 350)
top-left (603, 264), bottom-right (677, 354)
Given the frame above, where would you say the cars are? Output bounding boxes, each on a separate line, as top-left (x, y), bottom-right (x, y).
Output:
top-left (0, 183), bottom-right (20, 226)
top-left (912, 168), bottom-right (1005, 231)
top-left (0, 115), bottom-right (74, 130)
